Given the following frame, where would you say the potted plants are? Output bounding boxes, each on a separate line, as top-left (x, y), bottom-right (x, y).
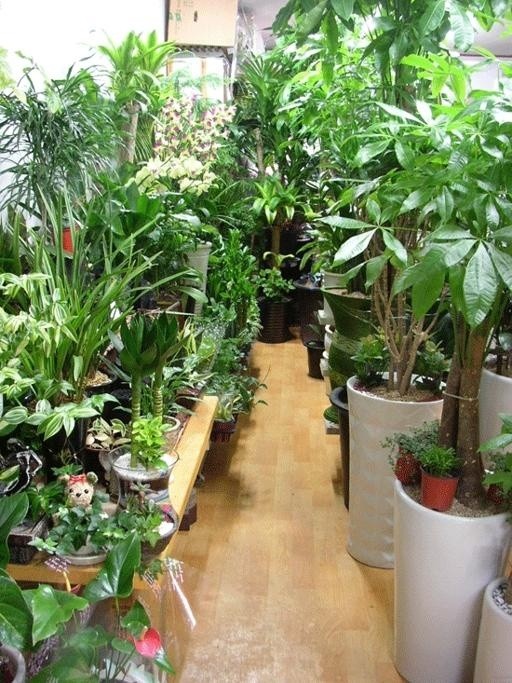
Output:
top-left (0, 2), bottom-right (512, 683)
